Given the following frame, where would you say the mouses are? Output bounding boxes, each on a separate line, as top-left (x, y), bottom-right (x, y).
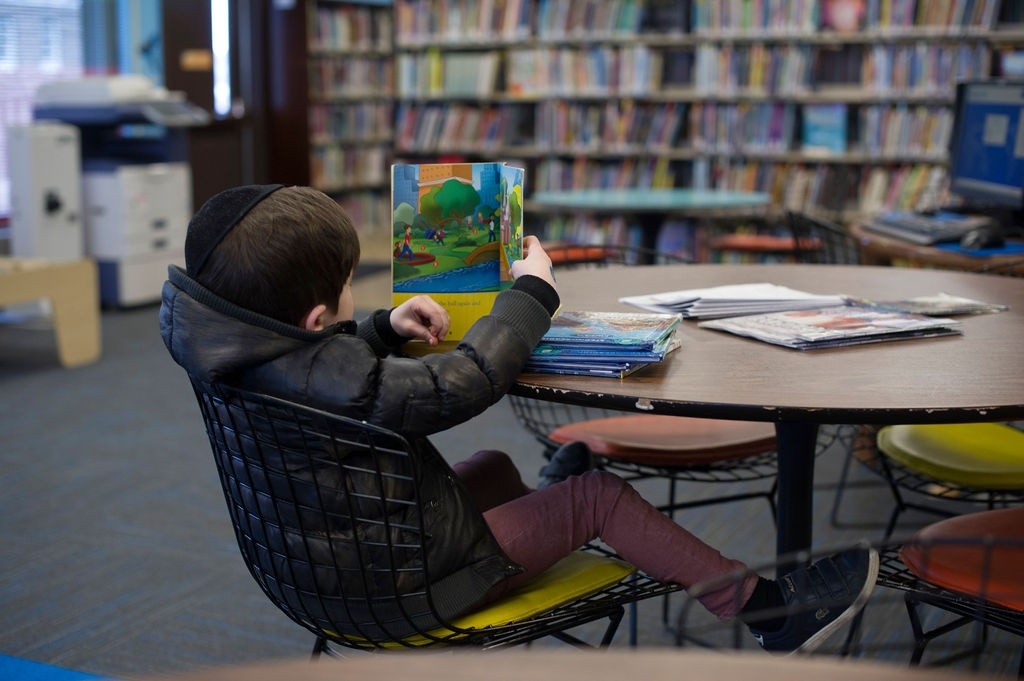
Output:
top-left (959, 225), bottom-right (1004, 249)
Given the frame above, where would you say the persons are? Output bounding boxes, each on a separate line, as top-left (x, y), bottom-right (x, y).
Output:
top-left (159, 184), bottom-right (879, 658)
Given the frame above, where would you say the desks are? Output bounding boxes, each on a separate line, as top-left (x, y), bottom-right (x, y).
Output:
top-left (838, 211), bottom-right (1024, 273)
top-left (531, 188), bottom-right (771, 266)
top-left (402, 263), bottom-right (1024, 580)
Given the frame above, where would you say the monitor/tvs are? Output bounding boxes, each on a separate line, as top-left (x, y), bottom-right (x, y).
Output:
top-left (948, 78), bottom-right (1024, 209)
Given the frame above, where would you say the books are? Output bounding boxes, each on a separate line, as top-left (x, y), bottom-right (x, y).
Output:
top-left (306, 0), bottom-right (1024, 266)
top-left (522, 311), bottom-right (682, 379)
top-left (391, 162), bottom-right (525, 342)
top-left (699, 291), bottom-right (1011, 351)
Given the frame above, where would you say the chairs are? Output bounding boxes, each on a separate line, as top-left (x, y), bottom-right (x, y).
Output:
top-left (189, 209), bottom-right (1024, 681)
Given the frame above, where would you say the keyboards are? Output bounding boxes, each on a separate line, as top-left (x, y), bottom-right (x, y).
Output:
top-left (859, 210), bottom-right (970, 247)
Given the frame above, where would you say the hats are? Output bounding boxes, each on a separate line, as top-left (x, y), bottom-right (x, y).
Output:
top-left (184, 183), bottom-right (283, 279)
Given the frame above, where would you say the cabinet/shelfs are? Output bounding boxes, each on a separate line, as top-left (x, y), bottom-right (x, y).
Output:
top-left (281, 0), bottom-right (1024, 267)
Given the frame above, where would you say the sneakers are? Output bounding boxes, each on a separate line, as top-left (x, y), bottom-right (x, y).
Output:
top-left (749, 541), bottom-right (881, 658)
top-left (537, 439), bottom-right (594, 492)
